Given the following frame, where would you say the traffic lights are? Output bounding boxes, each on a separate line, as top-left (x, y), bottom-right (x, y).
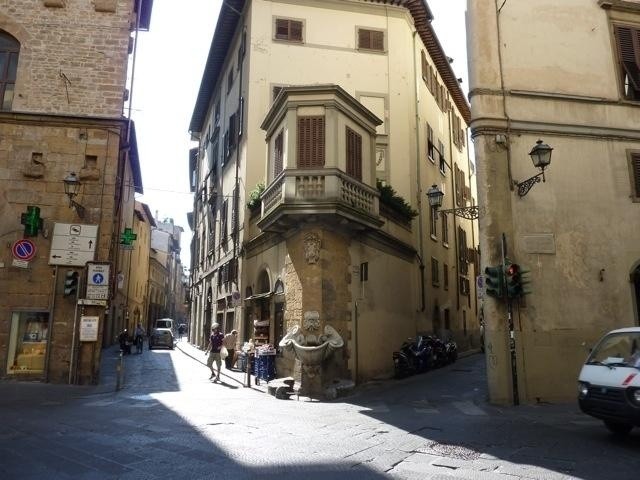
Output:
top-left (505, 263), bottom-right (523, 299)
top-left (484, 265), bottom-right (501, 297)
top-left (64, 269), bottom-right (80, 298)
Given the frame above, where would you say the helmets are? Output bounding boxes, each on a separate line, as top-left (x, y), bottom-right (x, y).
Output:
top-left (211, 322), bottom-right (219, 330)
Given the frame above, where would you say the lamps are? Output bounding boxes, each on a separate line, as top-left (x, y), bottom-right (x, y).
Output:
top-left (425, 182), bottom-right (480, 223)
top-left (517, 138), bottom-right (555, 201)
top-left (60, 170), bottom-right (86, 220)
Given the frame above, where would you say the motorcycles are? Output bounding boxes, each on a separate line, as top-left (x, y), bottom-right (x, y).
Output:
top-left (392, 333), bottom-right (458, 380)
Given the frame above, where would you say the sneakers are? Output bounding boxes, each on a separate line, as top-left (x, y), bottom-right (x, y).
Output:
top-left (209, 373), bottom-right (219, 381)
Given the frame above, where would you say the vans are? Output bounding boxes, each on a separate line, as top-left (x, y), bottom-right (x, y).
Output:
top-left (576, 324), bottom-right (640, 435)
top-left (154, 317), bottom-right (176, 336)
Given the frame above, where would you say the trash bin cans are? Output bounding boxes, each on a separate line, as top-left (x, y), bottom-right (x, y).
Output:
top-left (238, 346), bottom-right (276, 380)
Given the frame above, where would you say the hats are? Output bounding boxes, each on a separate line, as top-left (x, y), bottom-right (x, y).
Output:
top-left (231, 330), bottom-right (237, 334)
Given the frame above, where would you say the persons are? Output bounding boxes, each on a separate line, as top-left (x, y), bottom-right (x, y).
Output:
top-left (177, 324), bottom-right (185, 341)
top-left (133, 322), bottom-right (145, 355)
top-left (119, 328), bottom-right (129, 349)
top-left (231, 338), bottom-right (256, 368)
top-left (223, 329), bottom-right (238, 369)
top-left (204, 322), bottom-right (227, 383)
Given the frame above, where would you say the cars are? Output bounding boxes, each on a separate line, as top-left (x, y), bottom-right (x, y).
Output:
top-left (148, 327), bottom-right (175, 351)
